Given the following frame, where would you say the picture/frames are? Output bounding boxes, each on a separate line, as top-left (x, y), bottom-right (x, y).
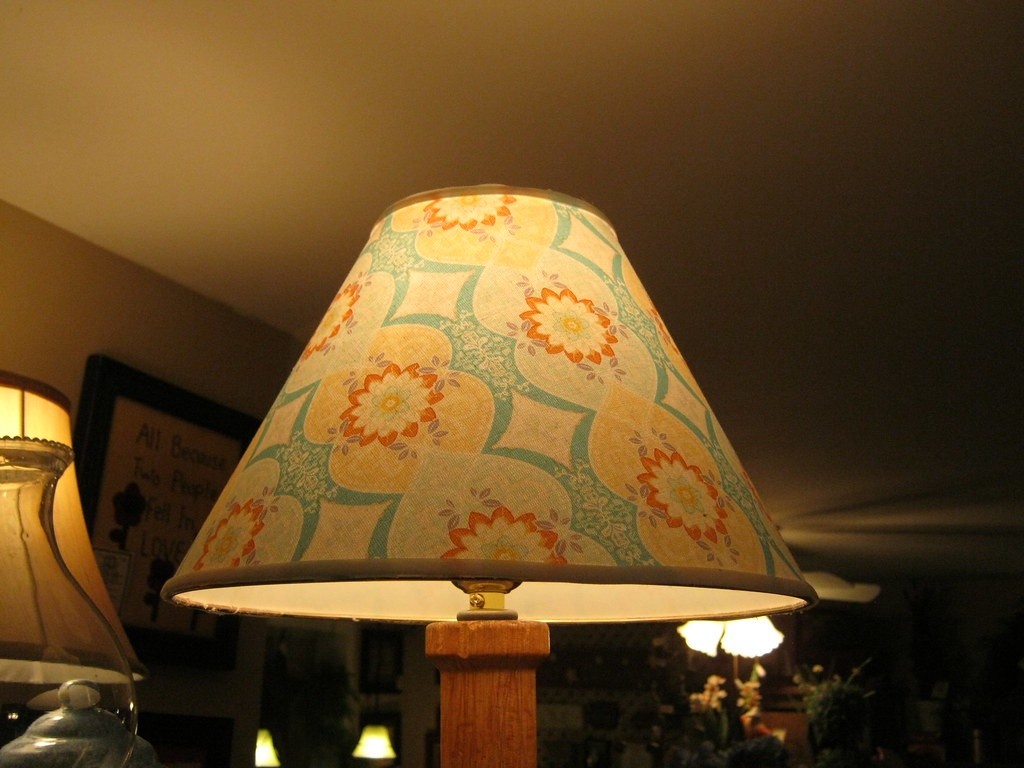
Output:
top-left (357, 625), bottom-right (406, 697)
top-left (358, 707), bottom-right (403, 765)
top-left (77, 352), bottom-right (262, 674)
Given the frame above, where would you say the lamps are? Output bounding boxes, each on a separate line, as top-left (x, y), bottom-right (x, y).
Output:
top-left (1, 370), bottom-right (145, 700)
top-left (674, 613), bottom-right (787, 659)
top-left (351, 726), bottom-right (398, 759)
top-left (162, 180), bottom-right (820, 768)
